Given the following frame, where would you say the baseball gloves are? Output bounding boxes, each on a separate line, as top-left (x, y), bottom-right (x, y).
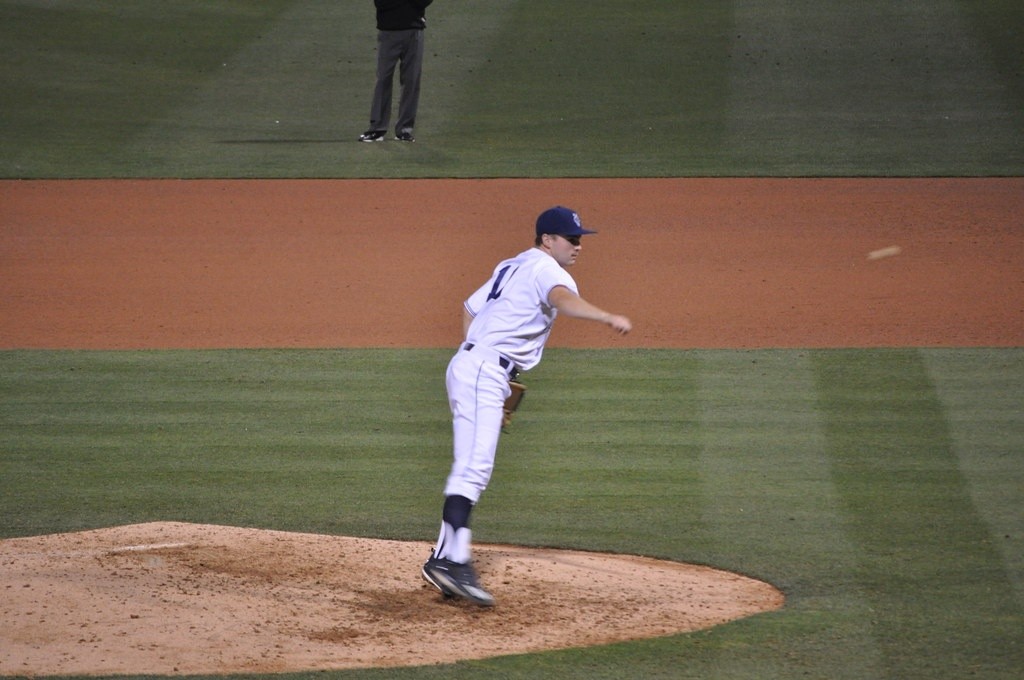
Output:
top-left (500, 379), bottom-right (527, 434)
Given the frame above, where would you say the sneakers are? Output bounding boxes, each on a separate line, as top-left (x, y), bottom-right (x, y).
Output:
top-left (359, 131), bottom-right (383, 142)
top-left (422, 552), bottom-right (495, 607)
top-left (394, 133), bottom-right (415, 143)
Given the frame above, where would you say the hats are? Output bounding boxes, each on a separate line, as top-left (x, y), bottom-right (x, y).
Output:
top-left (536, 206), bottom-right (597, 235)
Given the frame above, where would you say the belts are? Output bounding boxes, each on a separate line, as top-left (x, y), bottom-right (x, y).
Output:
top-left (463, 343), bottom-right (520, 378)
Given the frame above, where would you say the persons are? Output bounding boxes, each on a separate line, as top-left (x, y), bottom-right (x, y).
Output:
top-left (358, 0), bottom-right (433, 143)
top-left (422, 205), bottom-right (634, 606)
